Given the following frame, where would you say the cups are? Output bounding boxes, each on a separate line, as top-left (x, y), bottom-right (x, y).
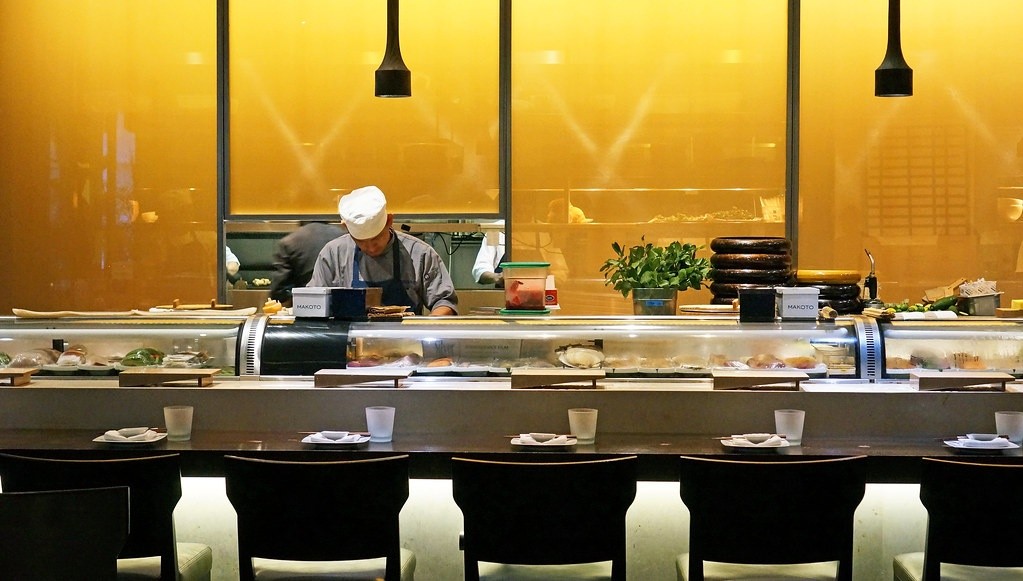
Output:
top-left (994, 411), bottom-right (1023, 445)
top-left (773, 409), bottom-right (804, 446)
top-left (365, 406), bottom-right (397, 443)
top-left (163, 405), bottom-right (194, 442)
top-left (567, 408), bottom-right (598, 445)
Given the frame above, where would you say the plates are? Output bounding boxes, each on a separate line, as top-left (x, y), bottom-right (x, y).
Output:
top-left (943, 434), bottom-right (1021, 452)
top-left (720, 433), bottom-right (790, 453)
top-left (510, 433), bottom-right (577, 450)
top-left (301, 431), bottom-right (371, 446)
top-left (558, 347), bottom-right (826, 371)
top-left (91, 427), bottom-right (168, 443)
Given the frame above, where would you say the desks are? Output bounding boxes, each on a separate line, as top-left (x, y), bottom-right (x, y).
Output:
top-left (0, 427), bottom-right (1023, 483)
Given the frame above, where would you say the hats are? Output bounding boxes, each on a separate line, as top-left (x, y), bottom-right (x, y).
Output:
top-left (339, 185), bottom-right (387, 240)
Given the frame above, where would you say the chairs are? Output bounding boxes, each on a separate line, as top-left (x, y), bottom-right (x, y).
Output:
top-left (451, 456), bottom-right (637, 581)
top-left (0, 453), bottom-right (212, 581)
top-left (893, 457), bottom-right (1023, 581)
top-left (676, 454), bottom-right (867, 581)
top-left (225, 455), bottom-right (416, 581)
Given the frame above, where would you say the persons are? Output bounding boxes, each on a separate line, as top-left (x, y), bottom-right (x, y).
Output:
top-left (305, 186), bottom-right (458, 317)
top-left (138, 189), bottom-right (240, 275)
top-left (269, 221), bottom-right (346, 304)
top-left (471, 219), bottom-right (569, 288)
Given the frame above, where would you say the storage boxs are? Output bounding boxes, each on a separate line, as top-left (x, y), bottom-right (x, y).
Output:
top-left (775, 287), bottom-right (820, 317)
top-left (228, 290), bottom-right (271, 310)
top-left (958, 293), bottom-right (1004, 316)
top-left (292, 288), bottom-right (383, 323)
top-left (737, 288), bottom-right (776, 323)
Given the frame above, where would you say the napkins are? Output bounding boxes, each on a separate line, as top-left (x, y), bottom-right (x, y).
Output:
top-left (312, 433), bottom-right (360, 442)
top-left (105, 430), bottom-right (157, 440)
top-left (520, 434), bottom-right (568, 443)
top-left (959, 437), bottom-right (1009, 447)
top-left (733, 436), bottom-right (781, 445)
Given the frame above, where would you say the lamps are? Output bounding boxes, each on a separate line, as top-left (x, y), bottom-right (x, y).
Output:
top-left (875, 0), bottom-right (913, 97)
top-left (375, 0), bottom-right (411, 97)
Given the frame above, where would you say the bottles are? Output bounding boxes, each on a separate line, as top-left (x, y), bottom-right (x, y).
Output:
top-left (864, 273), bottom-right (877, 300)
top-left (262, 297), bottom-right (282, 324)
